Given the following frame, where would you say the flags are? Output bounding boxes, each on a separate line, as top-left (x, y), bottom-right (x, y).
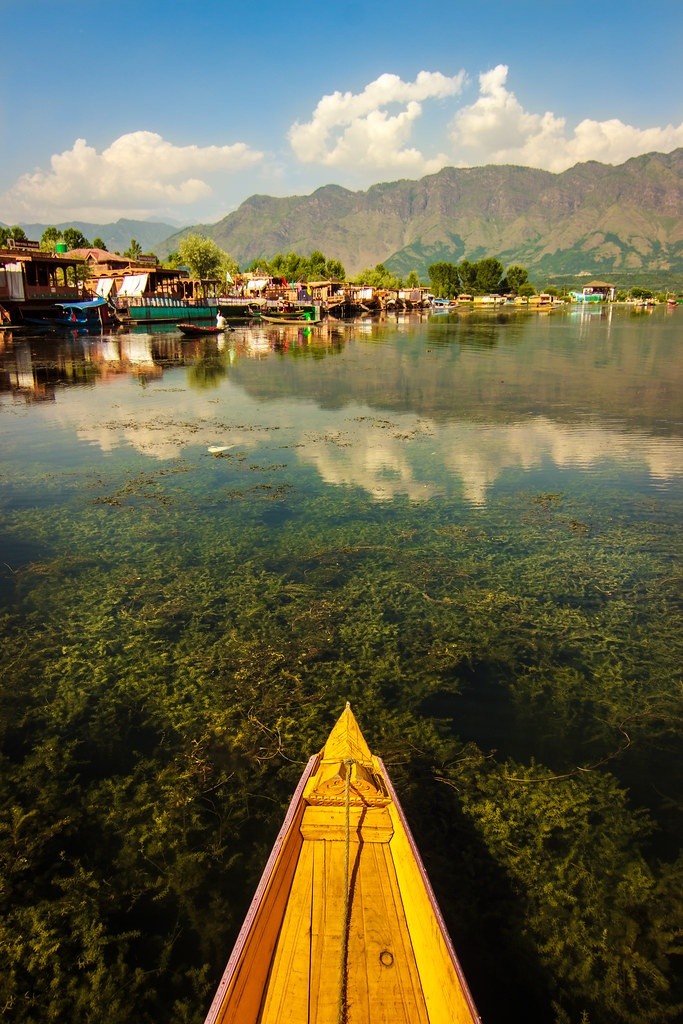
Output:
top-left (227, 271), bottom-right (312, 296)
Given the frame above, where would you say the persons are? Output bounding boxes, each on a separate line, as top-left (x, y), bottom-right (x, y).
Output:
top-left (216, 309), bottom-right (230, 330)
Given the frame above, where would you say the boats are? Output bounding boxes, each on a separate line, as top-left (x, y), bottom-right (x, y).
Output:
top-left (635, 302), bottom-right (656, 305)
top-left (529, 302), bottom-right (557, 311)
top-left (177, 324), bottom-right (230, 334)
top-left (204, 702), bottom-right (484, 1023)
top-left (21, 299), bottom-right (115, 327)
top-left (432, 300), bottom-right (460, 309)
top-left (668, 303), bottom-right (678, 307)
top-left (260, 315), bottom-right (323, 324)
